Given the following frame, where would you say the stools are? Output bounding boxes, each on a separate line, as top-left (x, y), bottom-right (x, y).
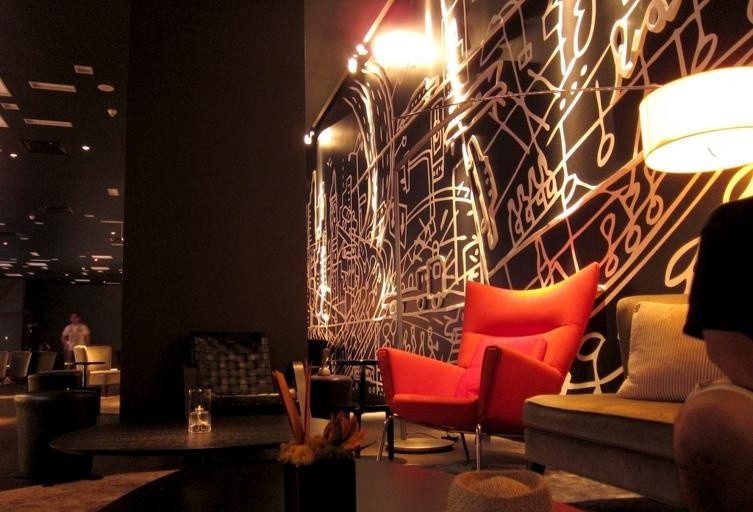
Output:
top-left (26, 369), bottom-right (83, 393)
top-left (15, 387), bottom-right (101, 477)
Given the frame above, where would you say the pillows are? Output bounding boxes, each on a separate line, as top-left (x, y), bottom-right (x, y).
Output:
top-left (615, 301), bottom-right (727, 403)
top-left (455, 335), bottom-right (547, 397)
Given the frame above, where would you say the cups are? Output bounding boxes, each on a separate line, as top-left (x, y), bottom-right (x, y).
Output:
top-left (185, 386), bottom-right (212, 435)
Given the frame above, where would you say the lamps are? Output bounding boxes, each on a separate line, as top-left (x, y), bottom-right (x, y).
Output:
top-left (639, 66), bottom-right (753, 174)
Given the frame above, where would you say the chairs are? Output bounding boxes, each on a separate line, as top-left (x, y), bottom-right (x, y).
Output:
top-left (183, 331), bottom-right (300, 417)
top-left (377, 261), bottom-right (600, 472)
top-left (64, 345), bottom-right (120, 397)
top-left (308, 339), bottom-right (328, 374)
top-left (0, 351), bottom-right (56, 385)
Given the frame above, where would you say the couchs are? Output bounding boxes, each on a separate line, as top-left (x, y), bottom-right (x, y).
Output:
top-left (521, 292), bottom-right (729, 509)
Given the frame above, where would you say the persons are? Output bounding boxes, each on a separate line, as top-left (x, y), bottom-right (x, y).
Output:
top-left (60, 312), bottom-right (91, 369)
top-left (672, 196), bottom-right (753, 512)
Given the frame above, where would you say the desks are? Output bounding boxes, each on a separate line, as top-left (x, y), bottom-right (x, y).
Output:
top-left (326, 359), bottom-right (394, 461)
top-left (48, 414), bottom-right (330, 512)
top-left (310, 373), bottom-right (354, 419)
